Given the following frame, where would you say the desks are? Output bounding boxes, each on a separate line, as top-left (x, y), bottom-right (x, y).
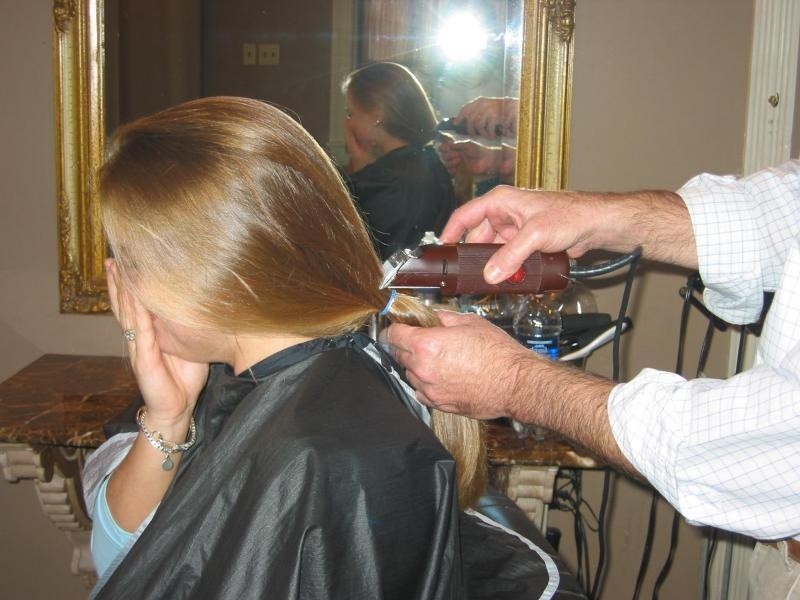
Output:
top-left (0, 353), bottom-right (606, 590)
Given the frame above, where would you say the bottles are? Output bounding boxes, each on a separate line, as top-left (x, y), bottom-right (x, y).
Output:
top-left (561, 278), bottom-right (599, 315)
top-left (511, 290), bottom-right (563, 441)
top-left (460, 293), bottom-right (511, 338)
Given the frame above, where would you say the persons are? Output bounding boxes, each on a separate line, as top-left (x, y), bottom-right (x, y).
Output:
top-left (337, 62), bottom-right (519, 261)
top-left (85, 95), bottom-right (488, 600)
top-left (377, 155), bottom-right (800, 600)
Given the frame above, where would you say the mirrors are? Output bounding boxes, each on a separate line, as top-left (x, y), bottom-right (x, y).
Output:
top-left (49, 0), bottom-right (576, 314)
